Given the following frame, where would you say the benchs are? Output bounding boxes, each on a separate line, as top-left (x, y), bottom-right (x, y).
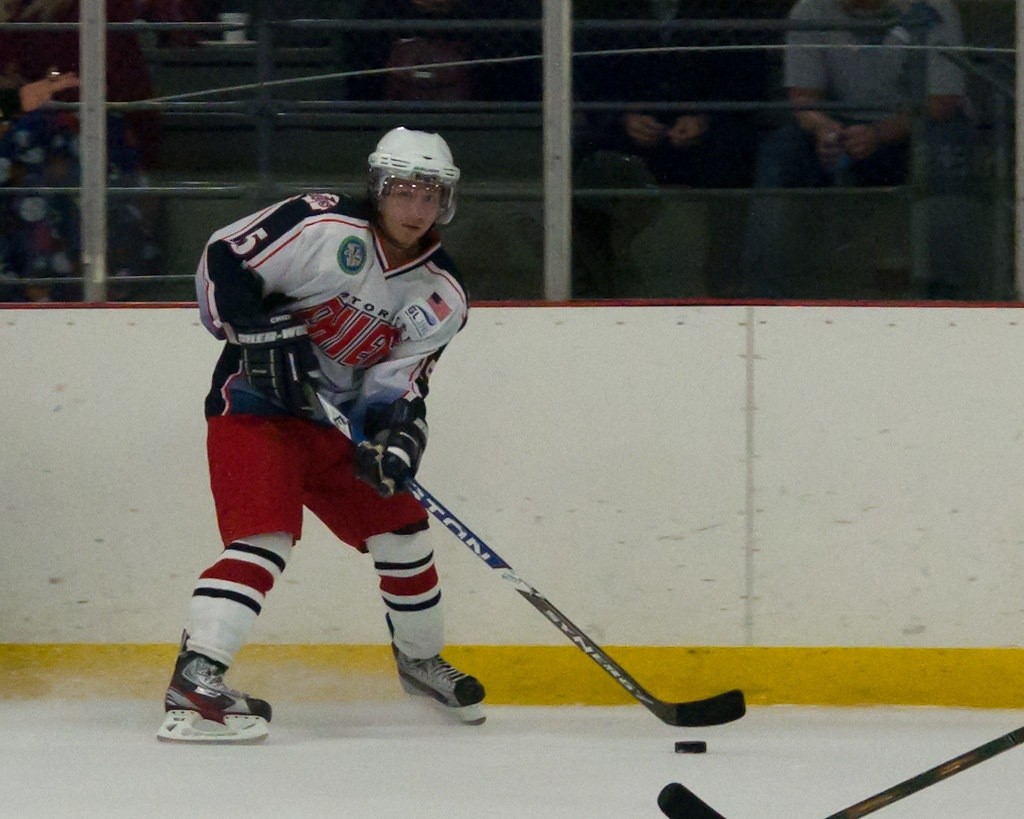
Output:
top-left (0, 0), bottom-right (1024, 306)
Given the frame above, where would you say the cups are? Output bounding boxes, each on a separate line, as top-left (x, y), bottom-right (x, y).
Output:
top-left (217, 10), bottom-right (251, 43)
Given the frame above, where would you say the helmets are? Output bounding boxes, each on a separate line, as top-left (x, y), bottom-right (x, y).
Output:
top-left (367, 127), bottom-right (460, 226)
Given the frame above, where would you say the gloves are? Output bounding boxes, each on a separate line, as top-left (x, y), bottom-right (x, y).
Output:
top-left (231, 311), bottom-right (321, 418)
top-left (355, 393), bottom-right (429, 496)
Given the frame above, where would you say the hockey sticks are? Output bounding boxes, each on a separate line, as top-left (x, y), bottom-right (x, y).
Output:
top-left (301, 382), bottom-right (746, 727)
top-left (656, 727), bottom-right (1024, 818)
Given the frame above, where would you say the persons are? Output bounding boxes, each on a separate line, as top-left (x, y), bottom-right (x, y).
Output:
top-left (383, 0), bottom-right (479, 106)
top-left (753, 0), bottom-right (968, 189)
top-left (0, 0), bottom-right (163, 186)
top-left (1, 70), bottom-right (82, 128)
top-left (580, 2), bottom-right (757, 188)
top-left (158, 125), bottom-right (487, 745)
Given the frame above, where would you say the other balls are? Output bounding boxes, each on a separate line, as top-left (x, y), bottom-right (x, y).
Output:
top-left (674, 740), bottom-right (707, 755)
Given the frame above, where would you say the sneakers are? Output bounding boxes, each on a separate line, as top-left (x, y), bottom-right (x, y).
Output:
top-left (391, 641), bottom-right (487, 726)
top-left (154, 628), bottom-right (273, 741)
